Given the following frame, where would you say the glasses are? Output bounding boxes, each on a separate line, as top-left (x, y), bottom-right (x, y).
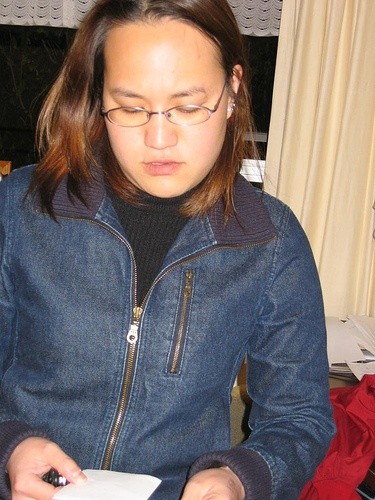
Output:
top-left (99, 79), bottom-right (229, 127)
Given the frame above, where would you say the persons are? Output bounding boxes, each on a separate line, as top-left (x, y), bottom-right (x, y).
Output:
top-left (0, 0), bottom-right (338, 500)
